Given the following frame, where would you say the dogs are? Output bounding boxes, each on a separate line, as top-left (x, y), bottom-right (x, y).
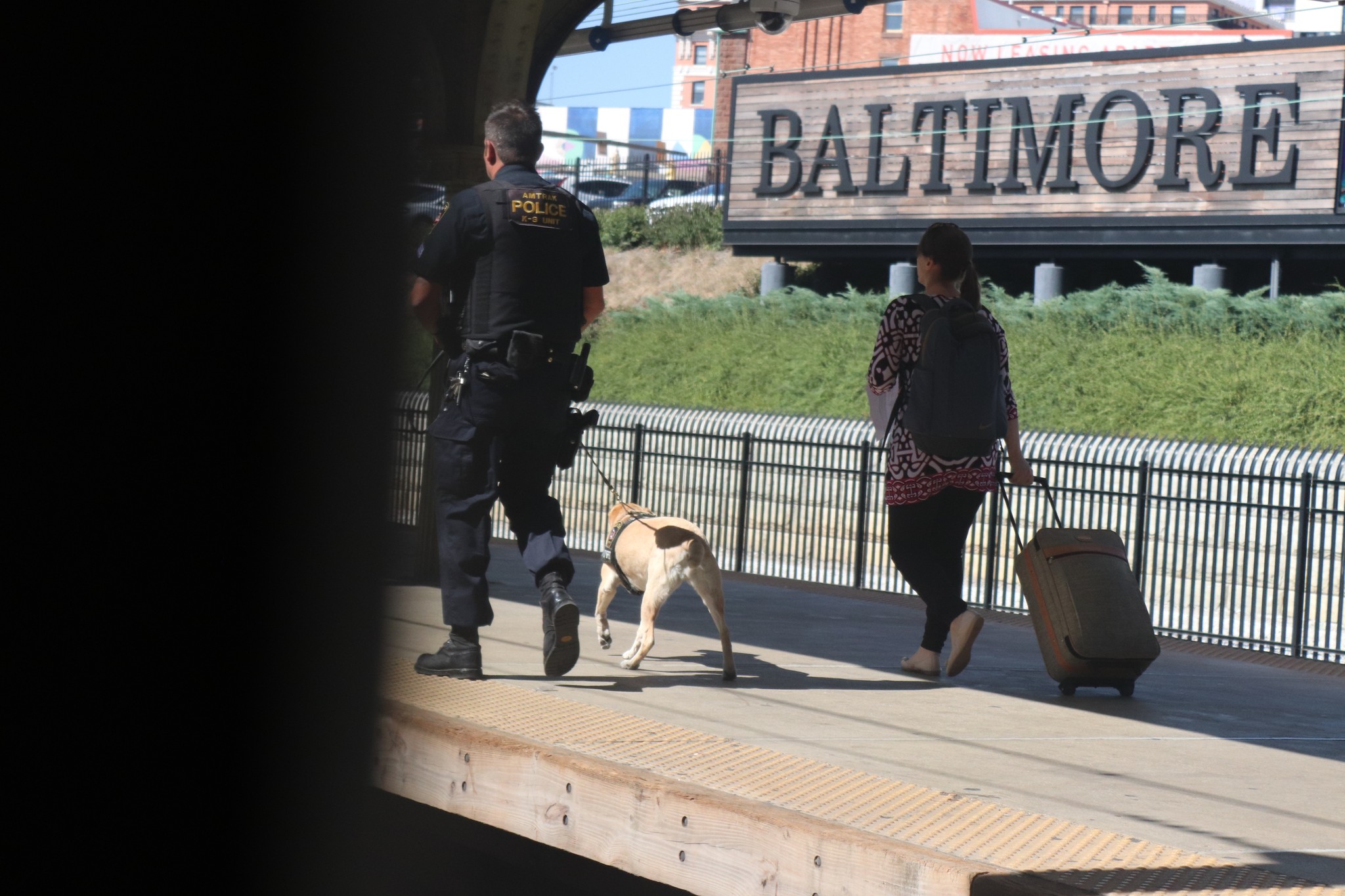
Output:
top-left (592, 501), bottom-right (739, 683)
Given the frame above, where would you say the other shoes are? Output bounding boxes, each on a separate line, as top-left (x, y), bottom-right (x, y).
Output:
top-left (945, 610), bottom-right (984, 677)
top-left (415, 629), bottom-right (485, 681)
top-left (536, 579), bottom-right (579, 680)
top-left (900, 653), bottom-right (942, 675)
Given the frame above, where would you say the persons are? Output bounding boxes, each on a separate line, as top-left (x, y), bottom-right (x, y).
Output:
top-left (410, 104), bottom-right (611, 679)
top-left (865, 222), bottom-right (1035, 678)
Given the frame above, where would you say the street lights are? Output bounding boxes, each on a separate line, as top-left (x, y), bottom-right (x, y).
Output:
top-left (550, 66), bottom-right (557, 105)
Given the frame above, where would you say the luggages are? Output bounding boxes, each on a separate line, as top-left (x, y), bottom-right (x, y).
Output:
top-left (996, 468), bottom-right (1158, 696)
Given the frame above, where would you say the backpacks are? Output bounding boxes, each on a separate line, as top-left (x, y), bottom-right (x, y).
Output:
top-left (896, 294), bottom-right (1009, 458)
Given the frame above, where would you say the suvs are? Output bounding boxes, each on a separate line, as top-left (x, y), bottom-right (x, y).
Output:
top-left (542, 171), bottom-right (726, 231)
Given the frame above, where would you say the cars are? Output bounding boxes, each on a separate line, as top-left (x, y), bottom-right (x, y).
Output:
top-left (406, 196), bottom-right (444, 227)
